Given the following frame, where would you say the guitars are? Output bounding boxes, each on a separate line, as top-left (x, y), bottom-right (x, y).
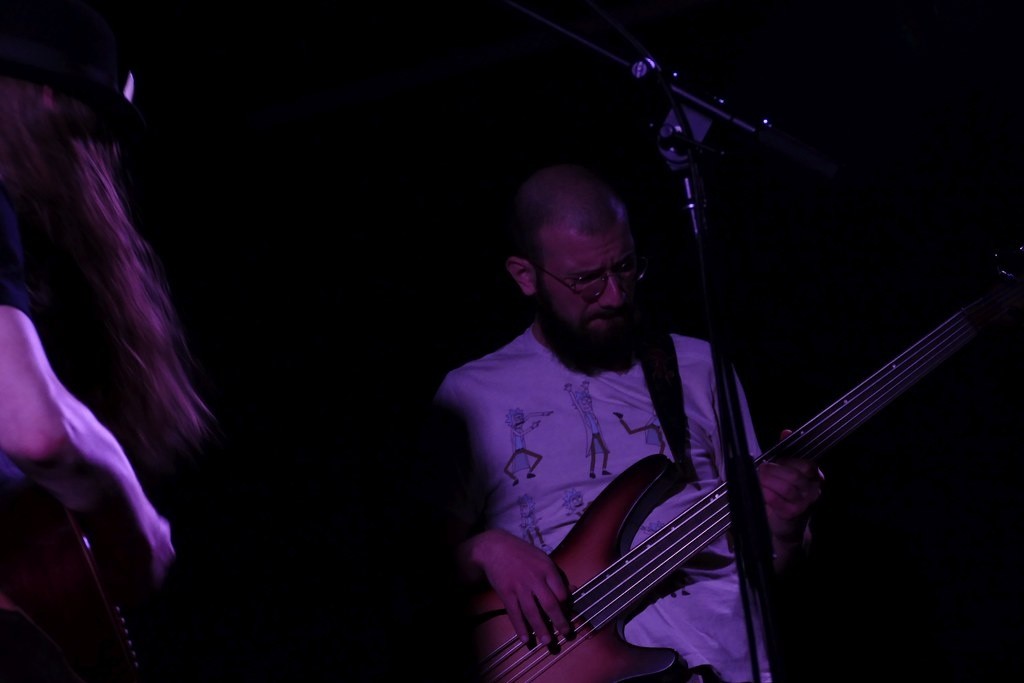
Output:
top-left (471, 245), bottom-right (1023, 683)
top-left (0, 480), bottom-right (139, 683)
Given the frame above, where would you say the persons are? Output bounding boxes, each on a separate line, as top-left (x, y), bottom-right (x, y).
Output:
top-left (0, 0), bottom-right (217, 583)
top-left (435, 166), bottom-right (824, 683)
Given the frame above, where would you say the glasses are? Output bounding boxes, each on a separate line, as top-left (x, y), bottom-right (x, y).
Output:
top-left (528, 243), bottom-right (650, 304)
